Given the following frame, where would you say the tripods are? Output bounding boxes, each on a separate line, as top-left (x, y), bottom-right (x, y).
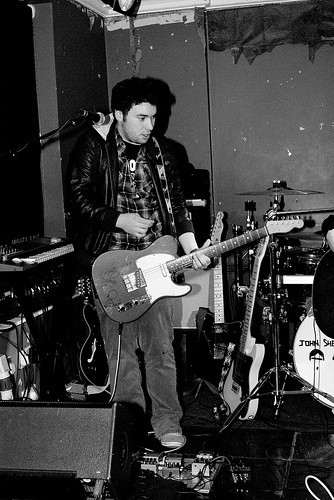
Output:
top-left (216, 222), bottom-right (334, 435)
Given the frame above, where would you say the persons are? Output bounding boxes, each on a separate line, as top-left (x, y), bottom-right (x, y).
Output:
top-left (322, 214), bottom-right (334, 253)
top-left (65, 77), bottom-right (210, 447)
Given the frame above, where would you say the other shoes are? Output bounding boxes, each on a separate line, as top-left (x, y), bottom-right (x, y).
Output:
top-left (161, 433), bottom-right (186, 453)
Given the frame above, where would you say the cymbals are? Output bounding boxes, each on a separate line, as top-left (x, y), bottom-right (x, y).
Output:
top-left (277, 231), bottom-right (332, 242)
top-left (234, 189), bottom-right (323, 195)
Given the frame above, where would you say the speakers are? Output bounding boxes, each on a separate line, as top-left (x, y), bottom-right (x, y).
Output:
top-left (1, 395), bottom-right (147, 483)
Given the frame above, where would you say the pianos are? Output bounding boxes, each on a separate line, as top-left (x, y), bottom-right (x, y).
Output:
top-left (1, 235), bottom-right (77, 271)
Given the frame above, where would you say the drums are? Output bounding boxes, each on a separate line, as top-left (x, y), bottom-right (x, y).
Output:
top-left (285, 249), bottom-right (326, 306)
top-left (292, 304), bottom-right (334, 410)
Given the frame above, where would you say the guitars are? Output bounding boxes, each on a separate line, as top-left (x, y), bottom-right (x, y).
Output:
top-left (89, 214), bottom-right (304, 325)
top-left (222, 232), bottom-right (266, 421)
top-left (196, 210), bottom-right (243, 390)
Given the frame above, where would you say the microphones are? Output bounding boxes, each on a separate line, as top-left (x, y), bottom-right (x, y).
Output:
top-left (79, 108), bottom-right (106, 126)
